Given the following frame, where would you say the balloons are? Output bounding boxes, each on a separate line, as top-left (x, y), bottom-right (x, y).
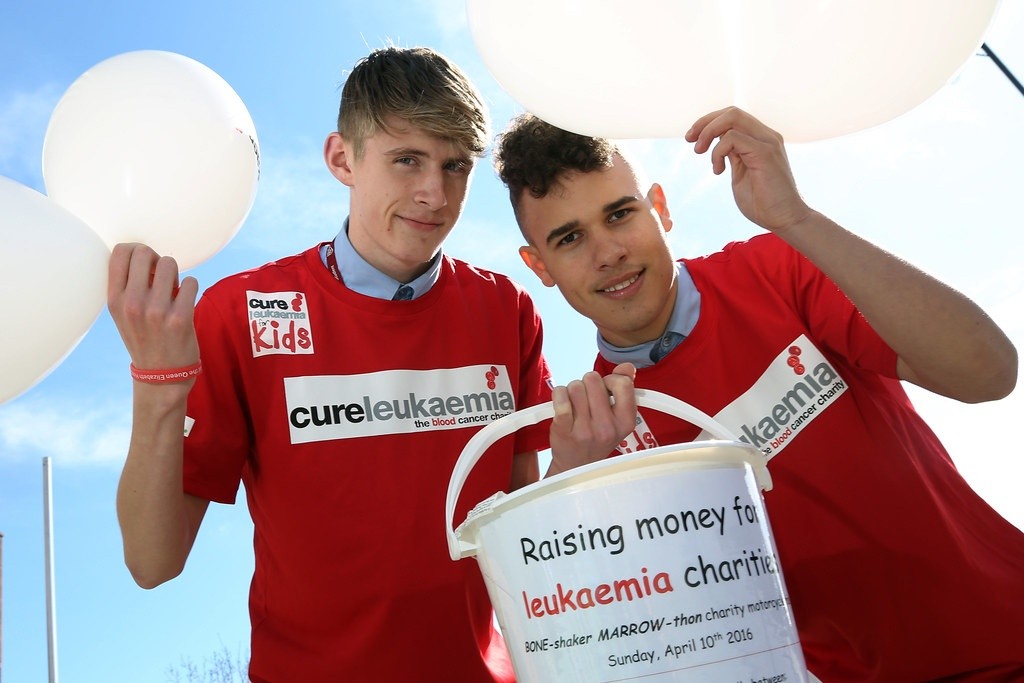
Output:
top-left (465, 0), bottom-right (997, 144)
top-left (40, 51), bottom-right (261, 275)
top-left (0, 177), bottom-right (110, 405)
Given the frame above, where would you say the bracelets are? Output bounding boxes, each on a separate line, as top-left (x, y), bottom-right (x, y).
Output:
top-left (131, 359), bottom-right (202, 384)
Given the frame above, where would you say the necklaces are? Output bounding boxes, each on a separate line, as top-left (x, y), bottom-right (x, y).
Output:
top-left (326, 237), bottom-right (345, 287)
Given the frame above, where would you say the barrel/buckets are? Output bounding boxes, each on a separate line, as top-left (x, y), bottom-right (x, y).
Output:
top-left (446, 389), bottom-right (813, 683)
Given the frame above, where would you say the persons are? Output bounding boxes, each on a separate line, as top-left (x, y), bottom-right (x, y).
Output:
top-left (107, 48), bottom-right (635, 683)
top-left (494, 106), bottom-right (1024, 683)
